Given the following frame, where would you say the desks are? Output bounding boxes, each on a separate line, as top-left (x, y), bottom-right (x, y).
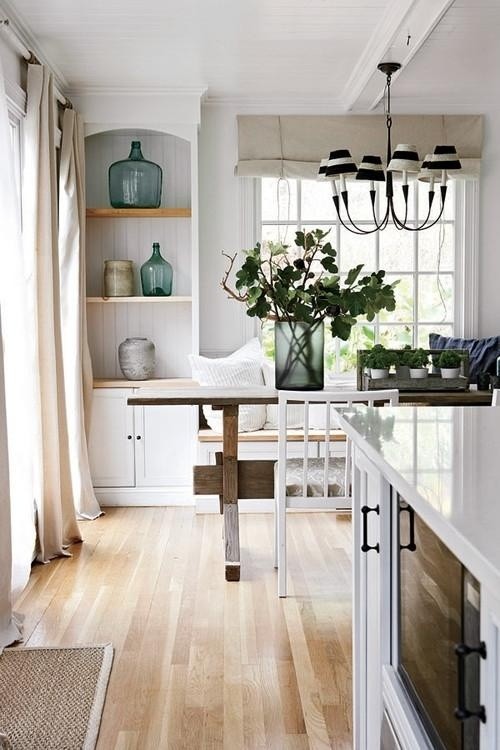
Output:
top-left (124, 384), bottom-right (499, 581)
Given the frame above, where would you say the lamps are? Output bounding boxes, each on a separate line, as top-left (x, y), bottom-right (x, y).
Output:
top-left (317, 63), bottom-right (464, 234)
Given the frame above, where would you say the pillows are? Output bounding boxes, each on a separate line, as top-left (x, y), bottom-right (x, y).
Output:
top-left (187, 337), bottom-right (266, 433)
top-left (429, 333), bottom-right (499, 383)
top-left (262, 360), bottom-right (344, 430)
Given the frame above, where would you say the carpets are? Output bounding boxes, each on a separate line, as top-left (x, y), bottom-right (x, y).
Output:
top-left (0, 643), bottom-right (115, 750)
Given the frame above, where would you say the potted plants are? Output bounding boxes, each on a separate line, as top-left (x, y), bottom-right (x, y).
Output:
top-left (433, 350), bottom-right (462, 380)
top-left (359, 344), bottom-right (409, 379)
top-left (402, 344), bottom-right (433, 379)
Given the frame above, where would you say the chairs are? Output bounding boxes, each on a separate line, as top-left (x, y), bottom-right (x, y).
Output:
top-left (269, 390), bottom-right (401, 598)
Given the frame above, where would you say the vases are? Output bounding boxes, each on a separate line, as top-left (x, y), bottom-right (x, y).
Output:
top-left (274, 318), bottom-right (325, 392)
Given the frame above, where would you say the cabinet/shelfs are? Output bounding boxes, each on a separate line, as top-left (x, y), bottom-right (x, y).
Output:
top-left (326, 404), bottom-right (499, 750)
top-left (75, 81), bottom-right (202, 508)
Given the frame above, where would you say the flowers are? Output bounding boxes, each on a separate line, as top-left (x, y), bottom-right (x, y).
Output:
top-left (219, 227), bottom-right (402, 387)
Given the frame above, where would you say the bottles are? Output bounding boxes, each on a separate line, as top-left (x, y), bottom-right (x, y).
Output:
top-left (141, 242), bottom-right (174, 297)
top-left (108, 140), bottom-right (162, 209)
top-left (104, 260), bottom-right (133, 297)
